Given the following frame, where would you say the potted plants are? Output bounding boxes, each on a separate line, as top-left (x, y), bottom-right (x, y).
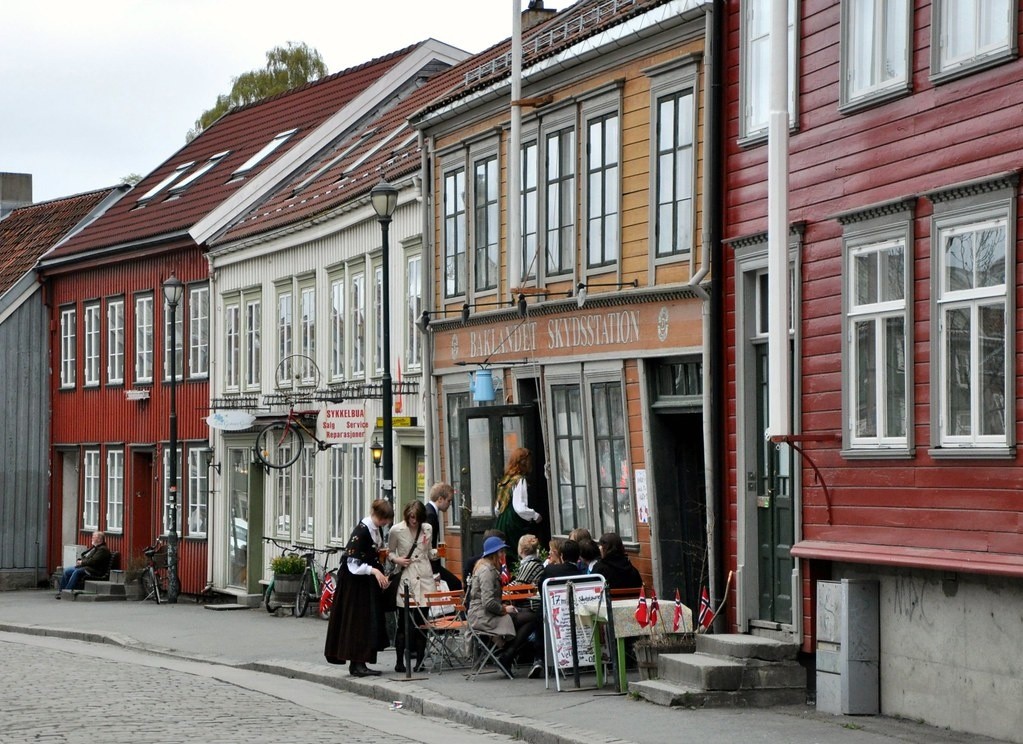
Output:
top-left (270, 556), bottom-right (315, 618)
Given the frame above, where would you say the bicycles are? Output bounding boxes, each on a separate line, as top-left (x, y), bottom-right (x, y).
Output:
top-left (262, 535), bottom-right (341, 619)
top-left (140, 535), bottom-right (165, 605)
top-left (255, 353), bottom-right (339, 469)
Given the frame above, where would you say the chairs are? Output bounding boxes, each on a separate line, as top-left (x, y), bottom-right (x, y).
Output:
top-left (398, 583), bottom-right (642, 682)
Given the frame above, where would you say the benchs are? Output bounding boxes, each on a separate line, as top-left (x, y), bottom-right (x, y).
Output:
top-left (51, 551), bottom-right (118, 602)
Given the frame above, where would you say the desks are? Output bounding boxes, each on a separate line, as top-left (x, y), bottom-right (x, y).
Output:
top-left (575, 598), bottom-right (694, 692)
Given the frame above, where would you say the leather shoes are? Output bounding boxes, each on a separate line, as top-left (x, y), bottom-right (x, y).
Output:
top-left (348, 665), bottom-right (381, 676)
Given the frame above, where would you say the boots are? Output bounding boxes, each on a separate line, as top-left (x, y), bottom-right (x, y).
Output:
top-left (494, 647), bottom-right (518, 678)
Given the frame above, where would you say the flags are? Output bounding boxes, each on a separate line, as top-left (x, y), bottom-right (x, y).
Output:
top-left (699, 588), bottom-right (715, 627)
top-left (636, 588), bottom-right (649, 628)
top-left (649, 589), bottom-right (659, 625)
top-left (673, 593), bottom-right (682, 630)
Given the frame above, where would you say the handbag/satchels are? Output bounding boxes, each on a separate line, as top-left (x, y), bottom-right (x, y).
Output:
top-left (380, 572), bottom-right (401, 613)
top-left (427, 572), bottom-right (455, 617)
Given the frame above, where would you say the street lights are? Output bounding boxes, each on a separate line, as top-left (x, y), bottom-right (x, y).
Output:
top-left (163, 269), bottom-right (186, 603)
top-left (368, 172), bottom-right (400, 531)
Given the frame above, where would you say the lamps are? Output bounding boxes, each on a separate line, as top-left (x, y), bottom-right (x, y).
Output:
top-left (578, 280), bottom-right (639, 308)
top-left (414, 309), bottom-right (462, 332)
top-left (461, 300), bottom-right (516, 327)
top-left (517, 290), bottom-right (574, 318)
top-left (204, 445), bottom-right (221, 476)
top-left (369, 437), bottom-right (384, 468)
top-left (250, 445), bottom-right (270, 475)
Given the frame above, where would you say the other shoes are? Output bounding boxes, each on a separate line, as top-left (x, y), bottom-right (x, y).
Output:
top-left (395, 663), bottom-right (406, 672)
top-left (414, 663), bottom-right (424, 672)
top-left (528, 659), bottom-right (543, 678)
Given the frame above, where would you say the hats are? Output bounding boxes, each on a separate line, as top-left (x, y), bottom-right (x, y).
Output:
top-left (482, 536), bottom-right (509, 557)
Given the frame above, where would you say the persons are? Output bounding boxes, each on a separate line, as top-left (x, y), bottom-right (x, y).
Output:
top-left (590, 532), bottom-right (642, 598)
top-left (570, 529), bottom-right (600, 574)
top-left (324, 499), bottom-right (393, 678)
top-left (466, 537), bottom-right (536, 678)
top-left (528, 541), bottom-right (584, 678)
top-left (388, 500), bottom-right (447, 674)
top-left (543, 538), bottom-right (565, 567)
top-left (494, 447), bottom-right (542, 561)
top-left (60, 533), bottom-right (110, 591)
top-left (484, 528), bottom-right (512, 585)
top-left (425, 482), bottom-right (462, 590)
top-left (503, 534), bottom-right (543, 610)
